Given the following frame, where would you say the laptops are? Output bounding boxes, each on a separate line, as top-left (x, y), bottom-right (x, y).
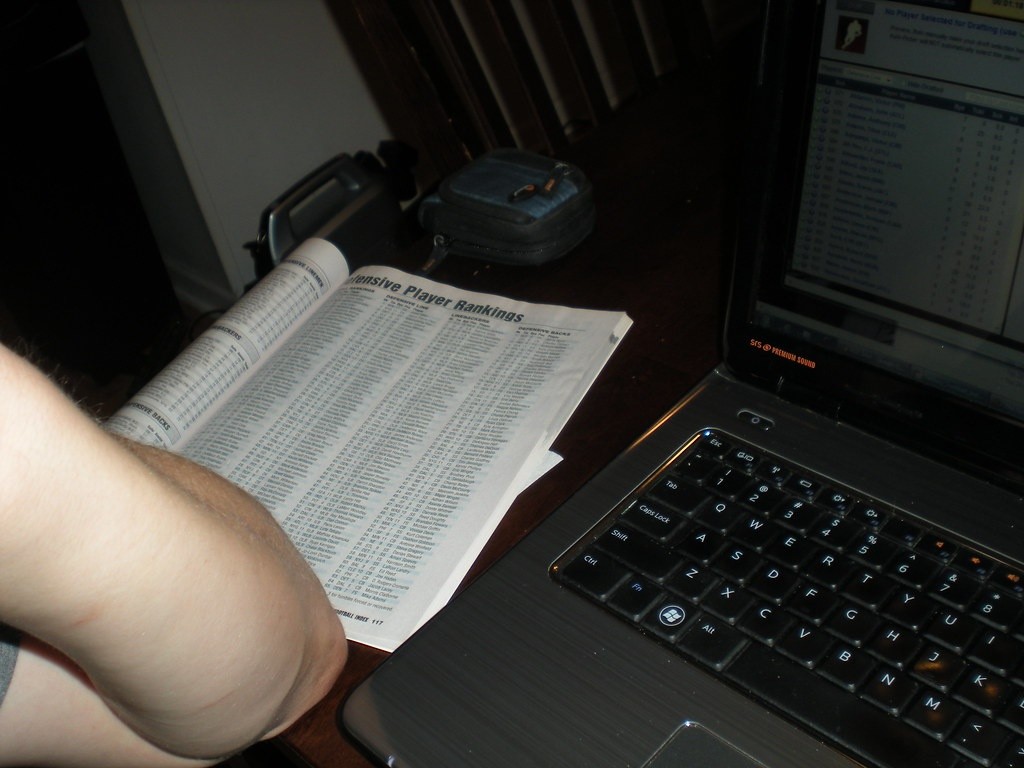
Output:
top-left (335, 3), bottom-right (1024, 768)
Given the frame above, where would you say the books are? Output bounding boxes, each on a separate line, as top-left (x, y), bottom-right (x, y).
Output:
top-left (99, 236), bottom-right (634, 655)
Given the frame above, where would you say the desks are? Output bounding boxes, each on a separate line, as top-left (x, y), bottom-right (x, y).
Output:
top-left (80, 143), bottom-right (749, 768)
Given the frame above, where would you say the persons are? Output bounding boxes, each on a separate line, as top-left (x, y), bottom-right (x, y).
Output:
top-left (0, 337), bottom-right (349, 768)
